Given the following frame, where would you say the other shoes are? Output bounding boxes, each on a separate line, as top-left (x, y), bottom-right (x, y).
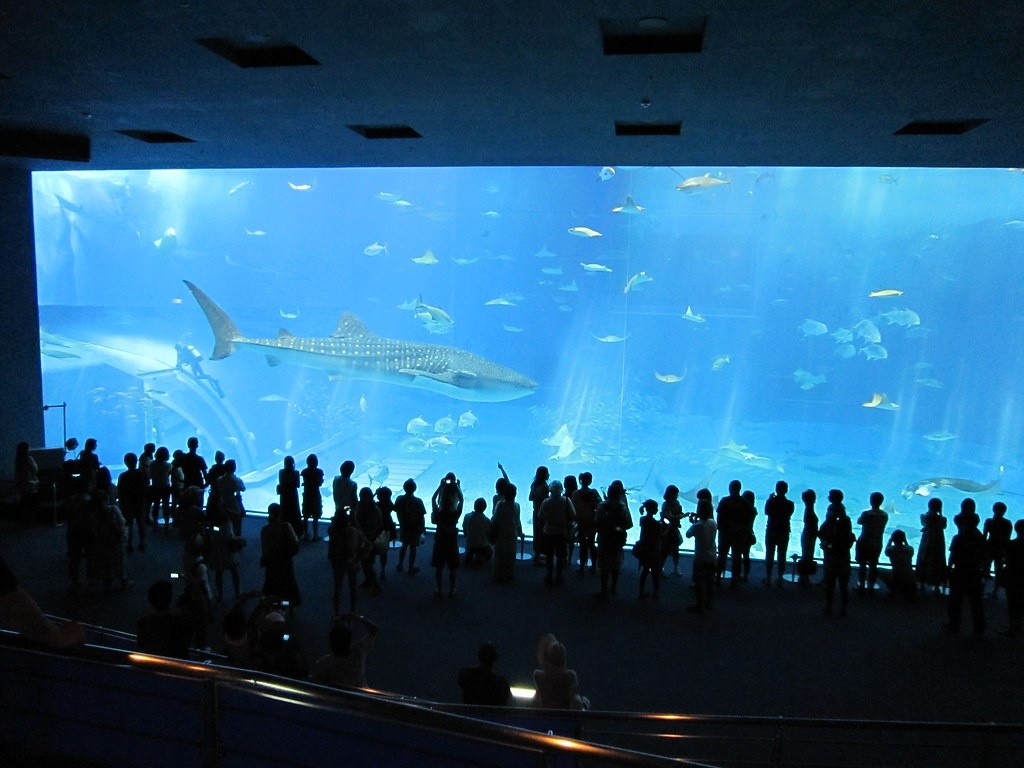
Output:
top-left (822, 606), bottom-right (833, 618)
top-left (448, 589), bottom-right (458, 598)
top-left (687, 604), bottom-right (707, 615)
top-left (408, 566), bottom-right (420, 575)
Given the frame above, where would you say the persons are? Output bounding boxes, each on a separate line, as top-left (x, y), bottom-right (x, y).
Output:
top-left (1, 437), bottom-right (1024, 711)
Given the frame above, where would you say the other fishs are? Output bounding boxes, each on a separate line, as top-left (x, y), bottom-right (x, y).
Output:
top-left (39, 165), bottom-right (1022, 522)
